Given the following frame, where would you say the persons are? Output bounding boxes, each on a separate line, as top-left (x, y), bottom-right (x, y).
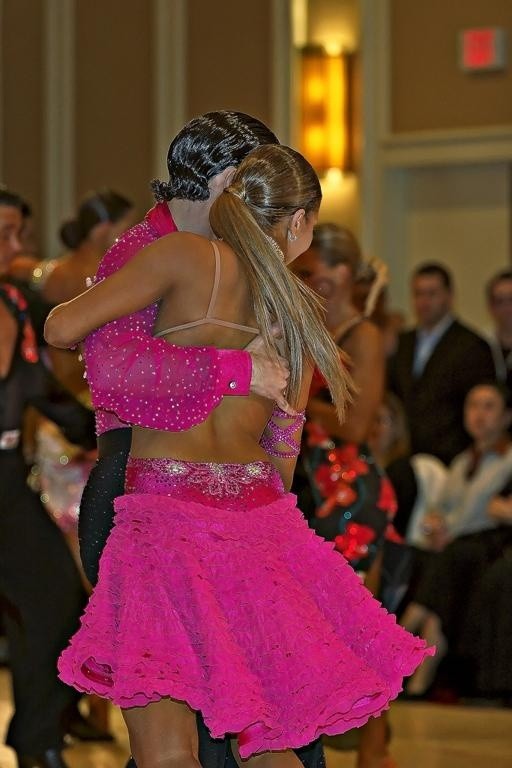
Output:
top-left (287, 222), bottom-right (511, 768)
top-left (79, 107), bottom-right (328, 767)
top-left (2, 188), bottom-right (154, 768)
top-left (48, 144), bottom-right (434, 767)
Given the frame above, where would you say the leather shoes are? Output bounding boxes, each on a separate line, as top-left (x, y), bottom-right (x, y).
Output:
top-left (69, 711), bottom-right (113, 743)
top-left (16, 748), bottom-right (66, 768)
